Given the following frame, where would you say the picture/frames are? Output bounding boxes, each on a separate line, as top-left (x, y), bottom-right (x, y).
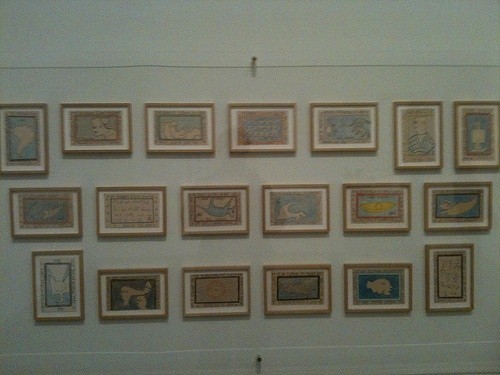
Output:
top-left (393, 100), bottom-right (443, 170)
top-left (262, 184), bottom-right (331, 233)
top-left (144, 102), bottom-right (216, 154)
top-left (424, 181), bottom-right (493, 231)
top-left (227, 103), bottom-right (297, 153)
top-left (0, 102), bottom-right (49, 175)
top-left (181, 267), bottom-right (251, 319)
top-left (424, 244), bottom-right (474, 313)
top-left (61, 102), bottom-right (132, 153)
top-left (310, 102), bottom-right (379, 153)
top-left (344, 263), bottom-right (413, 314)
top-left (342, 183), bottom-right (412, 233)
top-left (9, 187), bottom-right (82, 240)
top-left (180, 185), bottom-right (250, 235)
top-left (263, 265), bottom-right (333, 315)
top-left (97, 186), bottom-right (168, 237)
top-left (452, 100), bottom-right (500, 171)
top-left (31, 250), bottom-right (85, 323)
top-left (98, 269), bottom-right (169, 320)
